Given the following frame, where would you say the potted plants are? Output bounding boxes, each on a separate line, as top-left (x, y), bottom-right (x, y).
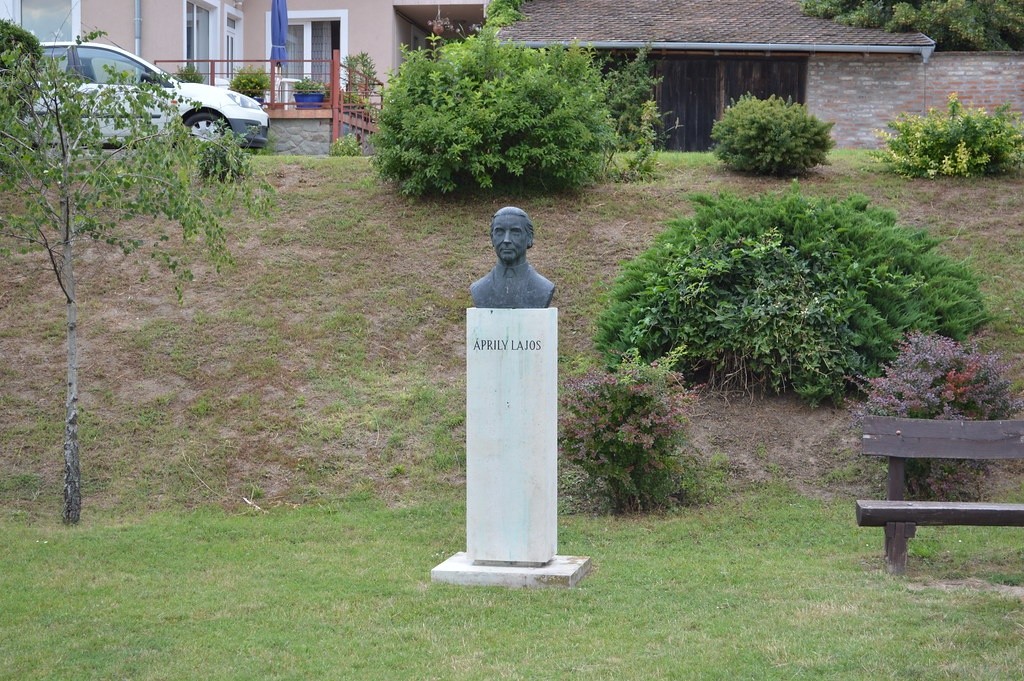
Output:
top-left (226, 64), bottom-right (271, 106)
top-left (294, 78), bottom-right (370, 120)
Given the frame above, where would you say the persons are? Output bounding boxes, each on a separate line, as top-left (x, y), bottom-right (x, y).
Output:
top-left (470, 207), bottom-right (555, 307)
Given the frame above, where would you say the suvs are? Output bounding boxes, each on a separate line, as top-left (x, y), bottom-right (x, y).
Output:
top-left (23, 40), bottom-right (270, 155)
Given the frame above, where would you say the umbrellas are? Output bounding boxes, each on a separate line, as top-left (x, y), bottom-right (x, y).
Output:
top-left (271, 0), bottom-right (288, 104)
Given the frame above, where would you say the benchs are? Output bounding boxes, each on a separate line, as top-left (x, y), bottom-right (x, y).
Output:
top-left (855, 416), bottom-right (1024, 574)
